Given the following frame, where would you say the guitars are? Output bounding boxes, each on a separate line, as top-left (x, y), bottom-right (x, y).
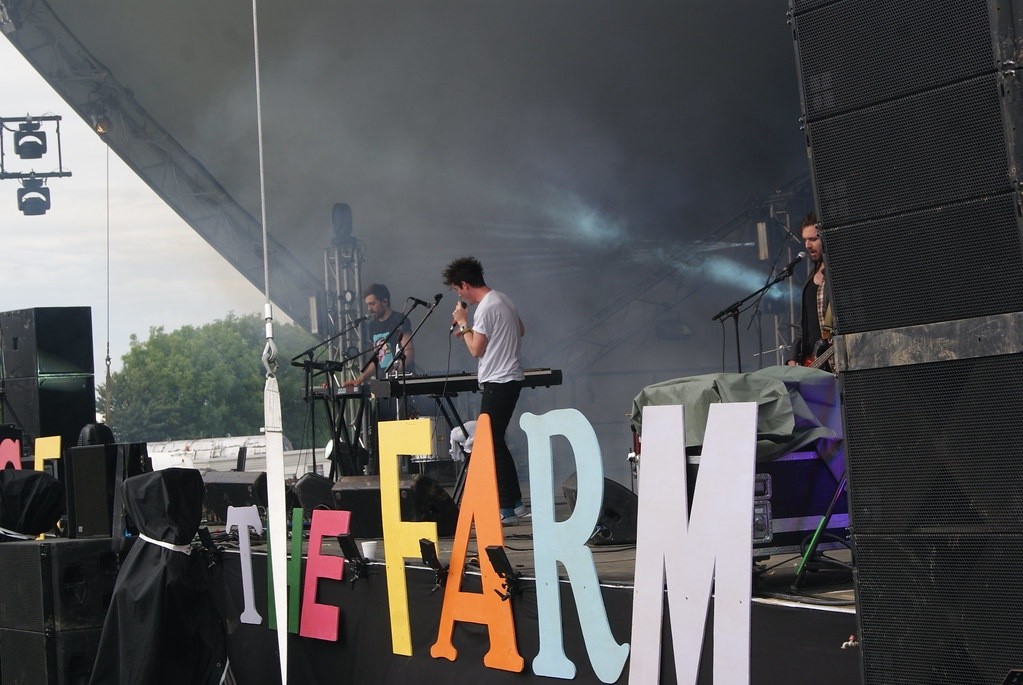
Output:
top-left (802, 337), bottom-right (835, 370)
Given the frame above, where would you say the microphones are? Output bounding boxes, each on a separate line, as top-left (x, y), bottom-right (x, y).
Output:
top-left (348, 313), bottom-right (371, 324)
top-left (450, 302), bottom-right (468, 333)
top-left (409, 296), bottom-right (431, 309)
top-left (394, 333), bottom-right (403, 370)
top-left (777, 251), bottom-right (806, 276)
top-left (783, 224), bottom-right (803, 244)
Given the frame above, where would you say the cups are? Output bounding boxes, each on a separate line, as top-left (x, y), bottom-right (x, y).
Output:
top-left (362, 541), bottom-right (378, 561)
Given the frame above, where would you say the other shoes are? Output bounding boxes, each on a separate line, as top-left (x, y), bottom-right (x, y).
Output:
top-left (513, 504), bottom-right (529, 518)
top-left (500, 515), bottom-right (517, 526)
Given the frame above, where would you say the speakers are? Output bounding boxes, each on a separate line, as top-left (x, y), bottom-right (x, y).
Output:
top-left (0, 537), bottom-right (138, 685)
top-left (331, 474), bottom-right (459, 538)
top-left (202, 471), bottom-right (292, 523)
top-left (62, 442), bottom-right (147, 538)
top-left (295, 472), bottom-right (335, 524)
top-left (785, 0), bottom-right (1023, 685)
top-left (0, 306), bottom-right (95, 380)
top-left (0, 375), bottom-right (96, 457)
top-left (561, 468), bottom-right (638, 545)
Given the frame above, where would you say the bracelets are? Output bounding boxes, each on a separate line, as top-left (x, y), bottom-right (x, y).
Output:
top-left (455, 324), bottom-right (471, 336)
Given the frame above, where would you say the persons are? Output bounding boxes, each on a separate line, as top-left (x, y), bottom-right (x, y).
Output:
top-left (785, 214), bottom-right (837, 374)
top-left (440, 257), bottom-right (526, 527)
top-left (343, 282), bottom-right (414, 476)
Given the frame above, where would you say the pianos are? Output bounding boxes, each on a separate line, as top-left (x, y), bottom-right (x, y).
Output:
top-left (382, 367), bottom-right (562, 505)
top-left (302, 383), bottom-right (373, 482)
